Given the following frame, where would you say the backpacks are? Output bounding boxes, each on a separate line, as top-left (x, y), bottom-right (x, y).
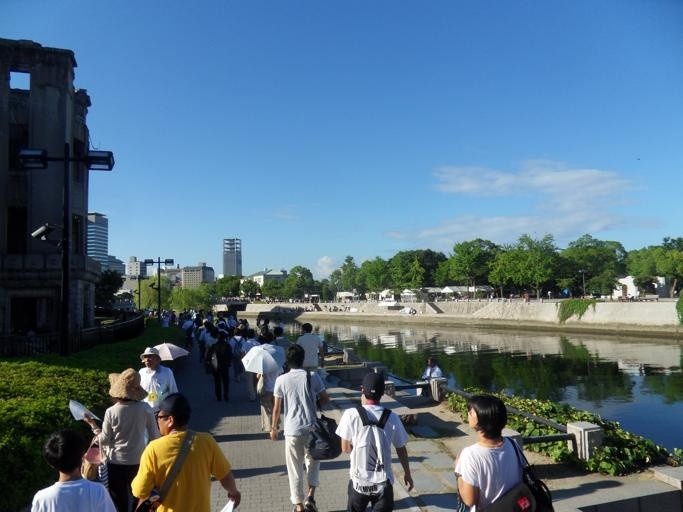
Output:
top-left (232, 336), bottom-right (245, 358)
top-left (351, 406), bottom-right (392, 497)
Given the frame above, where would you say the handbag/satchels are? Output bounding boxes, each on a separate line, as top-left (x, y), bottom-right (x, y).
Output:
top-left (210, 351), bottom-right (228, 376)
top-left (134, 487), bottom-right (161, 512)
top-left (478, 464), bottom-right (556, 511)
top-left (80, 447), bottom-right (110, 495)
top-left (308, 414), bottom-right (342, 460)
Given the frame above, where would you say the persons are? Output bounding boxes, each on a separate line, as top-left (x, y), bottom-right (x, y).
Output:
top-left (453, 395), bottom-right (524, 512)
top-left (421, 357), bottom-right (443, 381)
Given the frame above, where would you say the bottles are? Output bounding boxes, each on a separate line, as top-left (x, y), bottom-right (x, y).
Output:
top-left (137, 489), bottom-right (159, 511)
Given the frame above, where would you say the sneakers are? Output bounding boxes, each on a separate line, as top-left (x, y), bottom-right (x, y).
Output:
top-left (293, 496), bottom-right (319, 512)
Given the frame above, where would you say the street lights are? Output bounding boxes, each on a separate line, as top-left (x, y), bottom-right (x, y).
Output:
top-left (130, 275), bottom-right (150, 311)
top-left (144, 257), bottom-right (175, 325)
top-left (19, 140), bottom-right (115, 360)
top-left (578, 268), bottom-right (588, 296)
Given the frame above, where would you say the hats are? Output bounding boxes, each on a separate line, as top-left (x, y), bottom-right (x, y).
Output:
top-left (154, 393), bottom-right (191, 421)
top-left (362, 373), bottom-right (385, 393)
top-left (108, 368), bottom-right (148, 402)
top-left (140, 347), bottom-right (161, 363)
